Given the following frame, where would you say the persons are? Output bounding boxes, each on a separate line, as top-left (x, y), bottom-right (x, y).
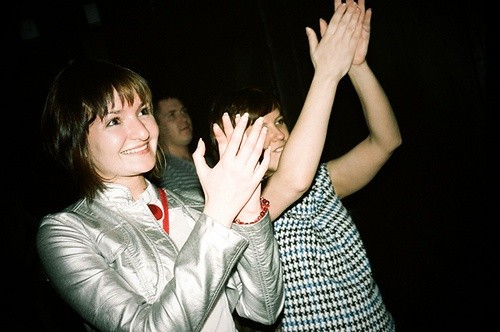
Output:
top-left (35, 54), bottom-right (286, 332)
top-left (209, 0), bottom-right (404, 332)
top-left (152, 96), bottom-right (215, 213)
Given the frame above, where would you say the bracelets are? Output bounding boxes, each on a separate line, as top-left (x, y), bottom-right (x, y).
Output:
top-left (232, 197), bottom-right (271, 225)
